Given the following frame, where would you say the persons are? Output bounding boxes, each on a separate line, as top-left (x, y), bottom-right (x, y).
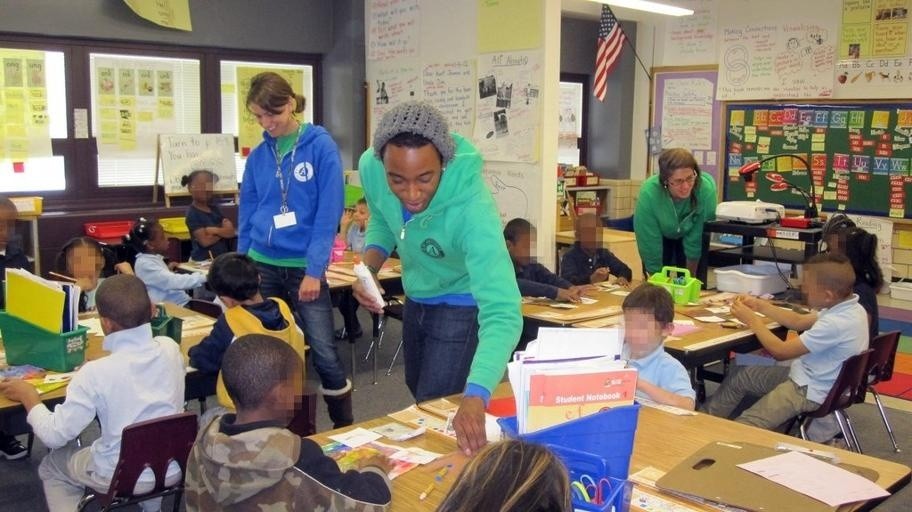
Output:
top-left (620, 282), bottom-right (696, 411)
top-left (179, 334), bottom-right (393, 512)
top-left (768, 227), bottom-right (885, 348)
top-left (352, 103), bottom-right (524, 455)
top-left (235, 72), bottom-right (353, 438)
top-left (560, 214), bottom-right (632, 287)
top-left (504, 219), bottom-right (583, 349)
top-left (188, 251), bottom-right (315, 433)
top-left (436, 439), bottom-right (573, 511)
top-left (0, 195), bottom-right (36, 460)
top-left (697, 253), bottom-right (870, 429)
top-left (337, 197), bottom-right (387, 340)
top-left (633, 148), bottom-right (718, 290)
top-left (0, 275), bottom-right (186, 512)
top-left (181, 170), bottom-right (235, 262)
top-left (121, 218), bottom-right (209, 310)
top-left (57, 236), bottom-right (135, 312)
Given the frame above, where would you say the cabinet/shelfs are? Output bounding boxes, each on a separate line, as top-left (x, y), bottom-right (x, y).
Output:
top-left (696, 211), bottom-right (827, 292)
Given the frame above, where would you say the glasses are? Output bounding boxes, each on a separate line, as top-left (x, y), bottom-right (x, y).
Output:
top-left (665, 175), bottom-right (697, 186)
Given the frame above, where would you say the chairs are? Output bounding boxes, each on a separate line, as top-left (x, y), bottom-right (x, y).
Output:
top-left (783, 347), bottom-right (880, 452)
top-left (75, 410), bottom-right (201, 512)
top-left (837, 330), bottom-right (903, 459)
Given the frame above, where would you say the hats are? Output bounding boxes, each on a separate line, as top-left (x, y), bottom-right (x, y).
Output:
top-left (372, 100), bottom-right (455, 170)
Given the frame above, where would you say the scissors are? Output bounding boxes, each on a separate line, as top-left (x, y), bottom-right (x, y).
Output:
top-left (586, 478), bottom-right (611, 505)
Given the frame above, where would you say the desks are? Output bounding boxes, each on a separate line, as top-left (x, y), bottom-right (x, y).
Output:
top-left (0, 324), bottom-right (311, 414)
top-left (569, 292), bottom-right (816, 402)
top-left (516, 277), bottom-right (712, 326)
top-left (323, 248), bottom-right (404, 281)
top-left (557, 220), bottom-right (640, 275)
top-left (300, 405), bottom-right (912, 512)
top-left (60, 299), bottom-right (216, 329)
top-left (414, 373), bottom-right (912, 512)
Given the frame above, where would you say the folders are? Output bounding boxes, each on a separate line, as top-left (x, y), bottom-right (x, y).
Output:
top-left (495, 399), bottom-right (643, 512)
top-left (0, 309), bottom-right (92, 373)
top-left (343, 184), bottom-right (366, 208)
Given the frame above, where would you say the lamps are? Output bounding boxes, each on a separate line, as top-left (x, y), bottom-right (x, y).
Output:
top-left (737, 153), bottom-right (828, 228)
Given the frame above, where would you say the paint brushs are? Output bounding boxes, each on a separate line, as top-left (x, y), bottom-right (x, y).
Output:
top-left (435, 466), bottom-right (453, 482)
top-left (418, 482), bottom-right (436, 500)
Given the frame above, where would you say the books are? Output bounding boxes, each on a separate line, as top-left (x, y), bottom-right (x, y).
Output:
top-left (506, 328), bottom-right (639, 435)
top-left (4, 268), bottom-right (82, 333)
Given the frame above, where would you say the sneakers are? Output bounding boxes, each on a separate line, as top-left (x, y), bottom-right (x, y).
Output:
top-left (1, 435), bottom-right (29, 460)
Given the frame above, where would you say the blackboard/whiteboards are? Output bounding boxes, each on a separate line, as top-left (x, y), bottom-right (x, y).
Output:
top-left (717, 97), bottom-right (912, 227)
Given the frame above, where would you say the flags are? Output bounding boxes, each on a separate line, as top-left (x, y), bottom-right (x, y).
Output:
top-left (592, 3), bottom-right (649, 101)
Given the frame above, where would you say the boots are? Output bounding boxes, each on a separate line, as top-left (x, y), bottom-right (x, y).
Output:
top-left (287, 388), bottom-right (318, 437)
top-left (318, 378), bottom-right (354, 429)
